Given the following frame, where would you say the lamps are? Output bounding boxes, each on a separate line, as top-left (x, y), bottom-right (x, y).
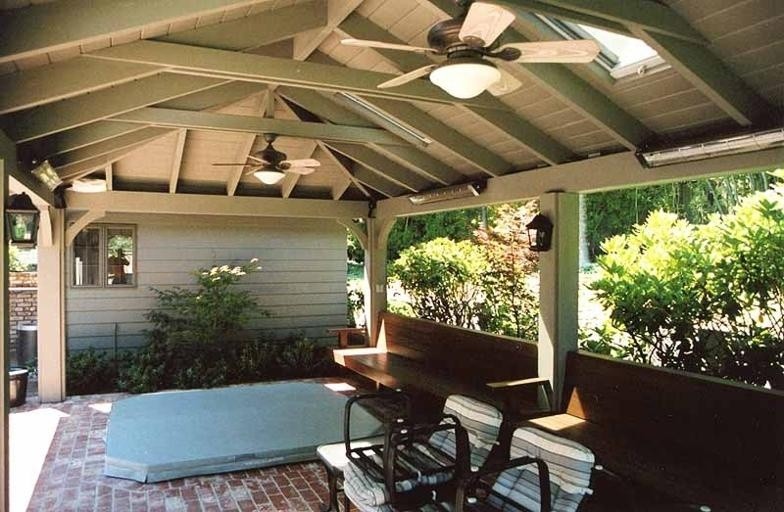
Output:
top-left (635, 116), bottom-right (784, 170)
top-left (23, 153), bottom-right (62, 194)
top-left (254, 165), bottom-right (285, 185)
top-left (409, 182), bottom-right (484, 206)
top-left (5, 192), bottom-right (39, 249)
top-left (526, 214), bottom-right (553, 252)
top-left (428, 49), bottom-right (500, 101)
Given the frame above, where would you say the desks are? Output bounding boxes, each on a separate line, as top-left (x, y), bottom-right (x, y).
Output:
top-left (316, 434), bottom-right (384, 511)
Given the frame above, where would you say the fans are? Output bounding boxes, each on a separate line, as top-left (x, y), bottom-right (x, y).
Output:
top-left (339, 0), bottom-right (601, 97)
top-left (212, 133), bottom-right (321, 176)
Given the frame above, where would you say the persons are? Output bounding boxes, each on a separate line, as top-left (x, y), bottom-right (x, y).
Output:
top-left (109, 248), bottom-right (129, 285)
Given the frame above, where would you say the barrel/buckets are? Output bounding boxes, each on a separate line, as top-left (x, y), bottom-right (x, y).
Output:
top-left (15, 320), bottom-right (37, 368)
top-left (8, 367), bottom-right (29, 407)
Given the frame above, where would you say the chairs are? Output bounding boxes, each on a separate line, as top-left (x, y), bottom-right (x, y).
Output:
top-left (343, 393), bottom-right (504, 511)
top-left (404, 426), bottom-right (596, 512)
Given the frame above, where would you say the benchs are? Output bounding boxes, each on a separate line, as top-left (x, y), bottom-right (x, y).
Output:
top-left (325, 311), bottom-right (538, 423)
top-left (521, 349), bottom-right (784, 511)
top-left (485, 467), bottom-right (521, 490)
